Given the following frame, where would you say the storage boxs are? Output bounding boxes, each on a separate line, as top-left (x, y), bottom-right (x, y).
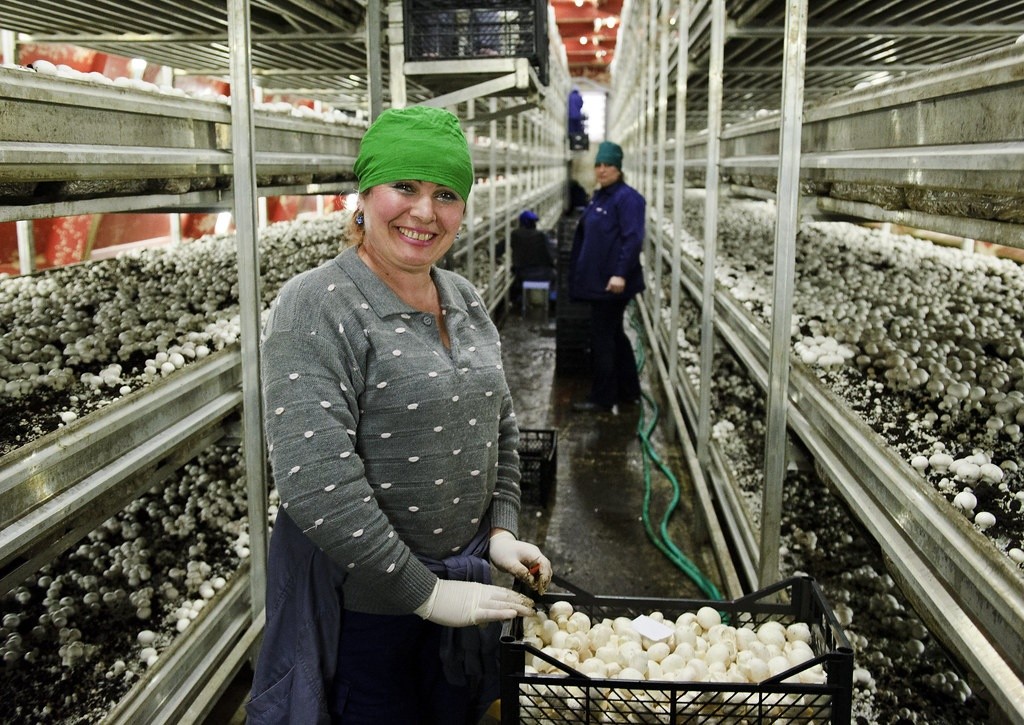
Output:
top-left (518, 428), bottom-right (559, 509)
top-left (400, 0), bottom-right (551, 85)
top-left (498, 574), bottom-right (854, 725)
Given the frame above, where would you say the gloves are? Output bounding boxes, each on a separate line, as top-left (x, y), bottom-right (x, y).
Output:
top-left (413, 576), bottom-right (535, 627)
top-left (489, 531), bottom-right (552, 596)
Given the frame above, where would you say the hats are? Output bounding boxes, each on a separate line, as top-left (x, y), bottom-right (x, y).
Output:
top-left (353, 106), bottom-right (474, 205)
top-left (596, 142), bottom-right (623, 169)
top-left (520, 212), bottom-right (539, 227)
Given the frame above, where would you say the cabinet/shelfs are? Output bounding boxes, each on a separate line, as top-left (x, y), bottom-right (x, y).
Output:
top-left (0, 0), bottom-right (573, 725)
top-left (603, 0), bottom-right (1024, 725)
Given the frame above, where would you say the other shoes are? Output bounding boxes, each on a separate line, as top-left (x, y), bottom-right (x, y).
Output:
top-left (573, 401), bottom-right (596, 410)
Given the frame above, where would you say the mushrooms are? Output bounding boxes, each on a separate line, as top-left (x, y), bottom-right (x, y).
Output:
top-left (519, 600), bottom-right (831, 725)
top-left (0, 58), bottom-right (368, 724)
top-left (651, 192), bottom-right (1024, 725)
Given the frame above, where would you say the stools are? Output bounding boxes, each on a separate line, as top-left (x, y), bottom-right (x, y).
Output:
top-left (521, 280), bottom-right (550, 323)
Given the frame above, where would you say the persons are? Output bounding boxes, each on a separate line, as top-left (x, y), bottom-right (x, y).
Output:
top-left (507, 212), bottom-right (557, 317)
top-left (568, 141), bottom-right (646, 412)
top-left (564, 179), bottom-right (588, 217)
top-left (568, 90), bottom-right (587, 149)
top-left (239, 105), bottom-right (554, 724)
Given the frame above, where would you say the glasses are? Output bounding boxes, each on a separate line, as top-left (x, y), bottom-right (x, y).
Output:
top-left (595, 163), bottom-right (612, 167)
